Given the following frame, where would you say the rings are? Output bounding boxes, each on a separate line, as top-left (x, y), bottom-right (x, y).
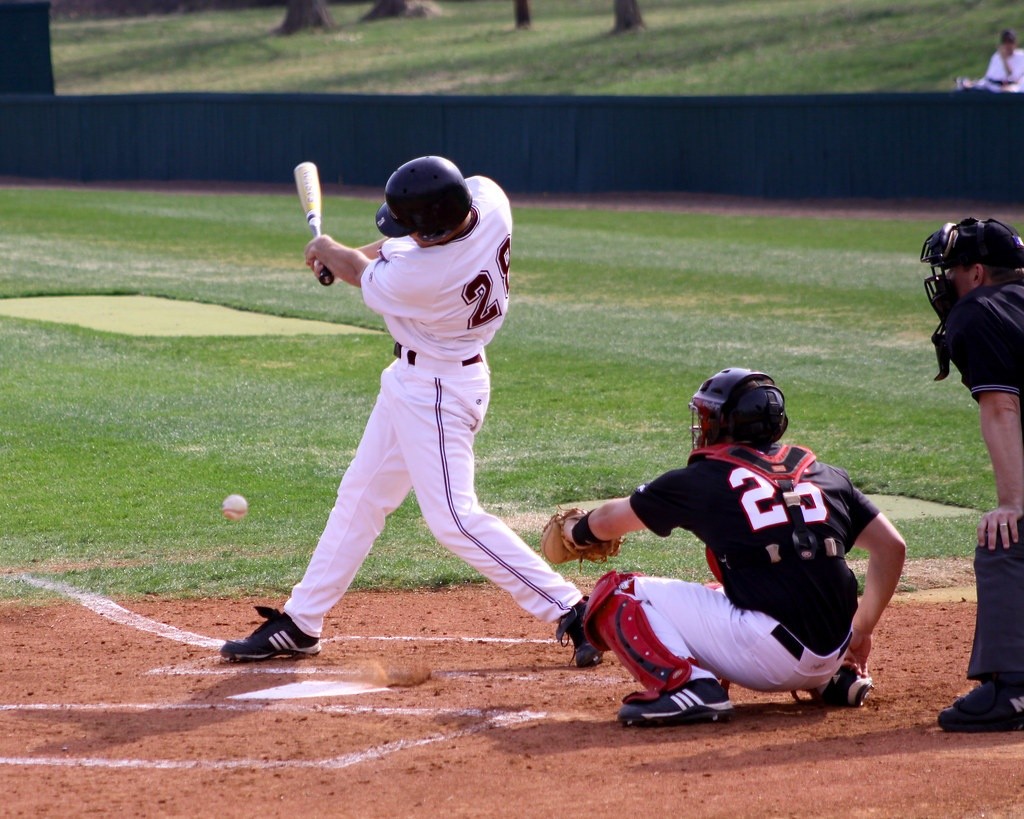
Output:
top-left (1000, 524), bottom-right (1007, 526)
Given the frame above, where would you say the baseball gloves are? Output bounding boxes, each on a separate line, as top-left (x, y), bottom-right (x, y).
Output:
top-left (541, 506), bottom-right (623, 565)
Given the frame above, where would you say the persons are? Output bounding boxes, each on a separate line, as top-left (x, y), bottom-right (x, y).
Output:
top-left (954, 30), bottom-right (1024, 94)
top-left (921, 217), bottom-right (1024, 733)
top-left (540, 367), bottom-right (905, 721)
top-left (218, 154), bottom-right (604, 667)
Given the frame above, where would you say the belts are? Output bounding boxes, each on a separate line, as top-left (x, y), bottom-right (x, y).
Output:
top-left (771, 625), bottom-right (853, 662)
top-left (393, 343), bottom-right (483, 368)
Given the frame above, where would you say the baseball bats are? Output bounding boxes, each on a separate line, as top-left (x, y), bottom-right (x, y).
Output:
top-left (295, 162), bottom-right (334, 287)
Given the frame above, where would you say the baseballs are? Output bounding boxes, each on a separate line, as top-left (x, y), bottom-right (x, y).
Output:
top-left (221, 494), bottom-right (248, 521)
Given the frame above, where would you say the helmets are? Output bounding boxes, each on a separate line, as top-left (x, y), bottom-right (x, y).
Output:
top-left (688, 367), bottom-right (788, 448)
top-left (945, 217), bottom-right (1024, 268)
top-left (375, 155), bottom-right (473, 241)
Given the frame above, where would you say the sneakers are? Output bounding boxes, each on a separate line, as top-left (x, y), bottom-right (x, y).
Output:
top-left (821, 664), bottom-right (876, 709)
top-left (219, 607), bottom-right (323, 662)
top-left (616, 677), bottom-right (735, 727)
top-left (556, 603), bottom-right (607, 667)
top-left (938, 682), bottom-right (1024, 733)
